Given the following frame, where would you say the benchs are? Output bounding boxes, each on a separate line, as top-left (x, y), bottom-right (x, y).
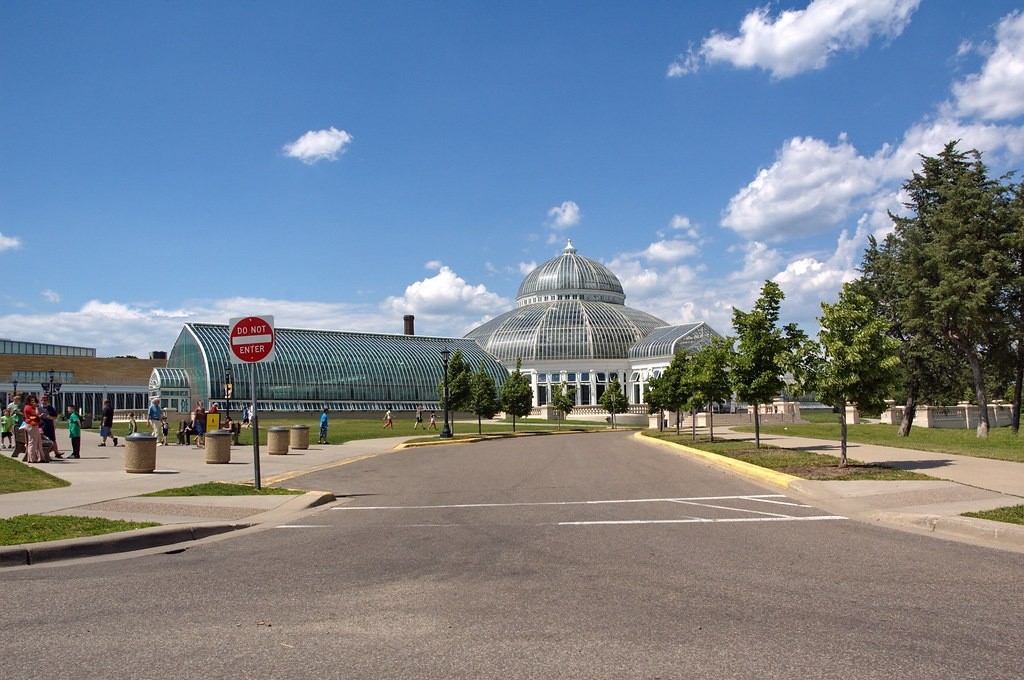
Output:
top-left (12, 425), bottom-right (55, 463)
top-left (179, 421), bottom-right (241, 446)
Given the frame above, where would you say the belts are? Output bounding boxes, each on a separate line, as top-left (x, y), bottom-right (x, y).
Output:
top-left (150, 418), bottom-right (159, 421)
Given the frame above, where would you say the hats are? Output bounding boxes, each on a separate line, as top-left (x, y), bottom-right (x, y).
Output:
top-left (212, 403), bottom-right (219, 407)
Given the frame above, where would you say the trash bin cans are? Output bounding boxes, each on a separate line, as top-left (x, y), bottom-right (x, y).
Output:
top-left (290, 425), bottom-right (309, 449)
top-left (124, 432), bottom-right (156, 474)
top-left (267, 427), bottom-right (290, 455)
top-left (204, 430), bottom-right (232, 464)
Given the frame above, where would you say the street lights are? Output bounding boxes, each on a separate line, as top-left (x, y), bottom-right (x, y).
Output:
top-left (440, 346), bottom-right (454, 438)
top-left (224, 366), bottom-right (231, 417)
top-left (40, 369), bottom-right (62, 407)
top-left (13, 380), bottom-right (18, 397)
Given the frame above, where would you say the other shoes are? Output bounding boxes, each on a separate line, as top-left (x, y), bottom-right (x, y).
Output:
top-left (98, 444), bottom-right (106, 446)
top-left (113, 438), bottom-right (117, 446)
top-left (67, 454), bottom-right (76, 459)
top-left (1, 444), bottom-right (6, 448)
top-left (8, 445), bottom-right (15, 448)
top-left (319, 441), bottom-right (330, 444)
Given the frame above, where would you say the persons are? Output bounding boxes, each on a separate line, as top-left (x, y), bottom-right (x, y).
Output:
top-left (221, 415), bottom-right (236, 445)
top-left (413, 406), bottom-right (426, 430)
top-left (146, 398), bottom-right (163, 447)
top-left (317, 408), bottom-right (330, 444)
top-left (241, 406), bottom-right (253, 428)
top-left (210, 402), bottom-right (219, 413)
top-left (161, 416), bottom-right (174, 446)
top-left (427, 410), bottom-right (440, 430)
top-left (179, 400), bottom-right (205, 447)
top-left (0, 393), bottom-right (65, 463)
top-left (97, 398), bottom-right (118, 446)
top-left (127, 412), bottom-right (137, 435)
top-left (66, 404), bottom-right (81, 458)
top-left (382, 406), bottom-right (396, 429)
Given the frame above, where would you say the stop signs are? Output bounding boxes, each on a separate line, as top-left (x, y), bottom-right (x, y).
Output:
top-left (229, 314), bottom-right (274, 364)
